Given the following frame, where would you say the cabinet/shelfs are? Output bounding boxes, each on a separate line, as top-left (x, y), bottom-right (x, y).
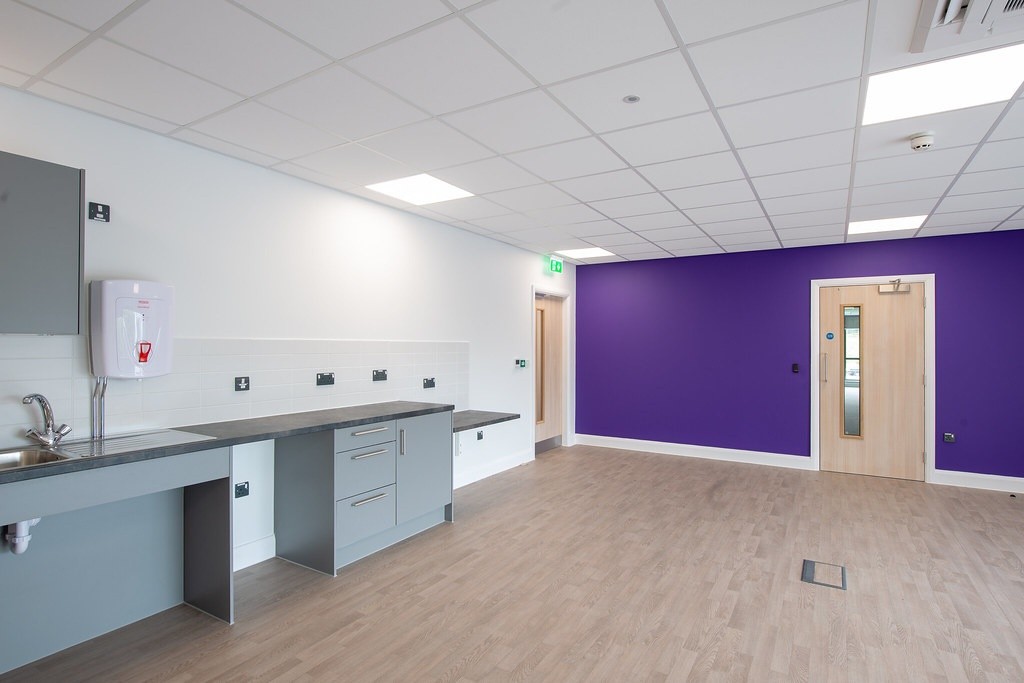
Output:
top-left (396, 410), bottom-right (452, 526)
top-left (0, 151), bottom-right (86, 336)
top-left (274, 419), bottom-right (397, 578)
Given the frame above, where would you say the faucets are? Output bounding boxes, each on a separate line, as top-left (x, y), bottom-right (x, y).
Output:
top-left (22, 392), bottom-right (71, 447)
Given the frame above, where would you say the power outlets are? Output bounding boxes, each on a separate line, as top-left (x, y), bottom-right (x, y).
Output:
top-left (944, 432), bottom-right (954, 442)
top-left (317, 373), bottom-right (334, 385)
top-left (235, 482), bottom-right (249, 499)
top-left (373, 370), bottom-right (387, 381)
top-left (89, 202), bottom-right (110, 222)
top-left (477, 431), bottom-right (483, 440)
top-left (235, 377), bottom-right (249, 391)
top-left (423, 378), bottom-right (435, 389)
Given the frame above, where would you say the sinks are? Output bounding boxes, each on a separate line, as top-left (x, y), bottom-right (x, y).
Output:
top-left (1, 448), bottom-right (70, 469)
